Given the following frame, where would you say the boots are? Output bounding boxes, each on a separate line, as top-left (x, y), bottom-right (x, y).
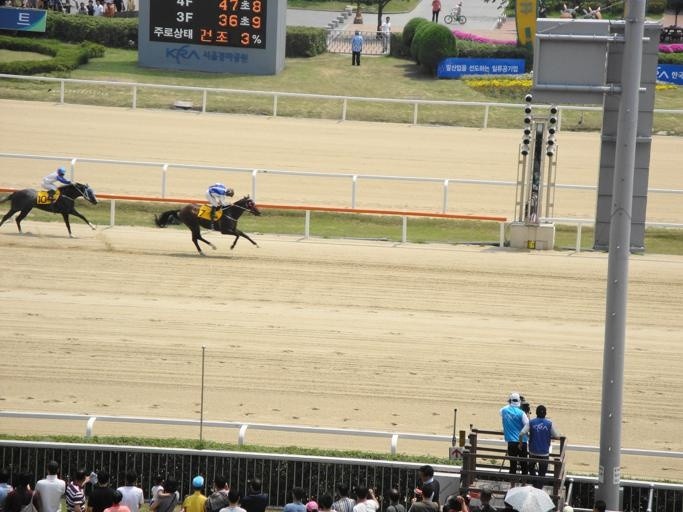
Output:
top-left (47, 190), bottom-right (57, 202)
top-left (211, 205), bottom-right (219, 218)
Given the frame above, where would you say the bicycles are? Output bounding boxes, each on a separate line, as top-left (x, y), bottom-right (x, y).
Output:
top-left (443, 9), bottom-right (467, 25)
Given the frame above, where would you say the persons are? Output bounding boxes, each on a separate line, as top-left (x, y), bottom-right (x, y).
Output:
top-left (39, 167), bottom-right (70, 201)
top-left (1, 460), bottom-right (574, 512)
top-left (454, 2), bottom-right (462, 20)
top-left (593, 500), bottom-right (606, 511)
top-left (351, 30), bottom-right (363, 65)
top-left (528, 405), bottom-right (559, 477)
top-left (431, 0), bottom-right (441, 23)
top-left (518, 396), bottom-right (531, 417)
top-left (0, 1), bottom-right (135, 20)
top-left (378, 17), bottom-right (392, 53)
top-left (499, 392), bottom-right (530, 475)
top-left (204, 183), bottom-right (234, 222)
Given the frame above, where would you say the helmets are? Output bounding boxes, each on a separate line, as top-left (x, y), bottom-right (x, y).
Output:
top-left (57, 166), bottom-right (66, 176)
top-left (225, 188), bottom-right (234, 197)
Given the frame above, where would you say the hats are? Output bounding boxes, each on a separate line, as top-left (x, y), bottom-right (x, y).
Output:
top-left (509, 392), bottom-right (521, 405)
top-left (192, 475), bottom-right (204, 489)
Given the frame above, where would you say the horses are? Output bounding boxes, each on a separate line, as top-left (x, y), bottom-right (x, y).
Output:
top-left (0, 181), bottom-right (100, 238)
top-left (153, 194), bottom-right (261, 257)
